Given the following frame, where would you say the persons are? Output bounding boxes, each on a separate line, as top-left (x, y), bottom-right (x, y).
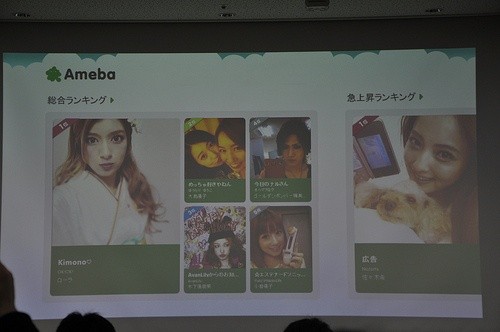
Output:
top-left (285, 317), bottom-right (333, 332)
top-left (57, 312), bottom-right (116, 332)
top-left (249, 209), bottom-right (306, 270)
top-left (0, 311), bottom-right (41, 332)
top-left (259, 120), bottom-right (311, 179)
top-left (52, 118), bottom-right (171, 245)
top-left (398, 114), bottom-right (481, 245)
top-left (185, 130), bottom-right (232, 178)
top-left (215, 119), bottom-right (245, 178)
top-left (204, 225), bottom-right (246, 272)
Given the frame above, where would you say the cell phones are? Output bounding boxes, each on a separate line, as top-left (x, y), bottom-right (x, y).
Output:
top-left (282, 227), bottom-right (298, 266)
top-left (353, 119), bottom-right (401, 179)
